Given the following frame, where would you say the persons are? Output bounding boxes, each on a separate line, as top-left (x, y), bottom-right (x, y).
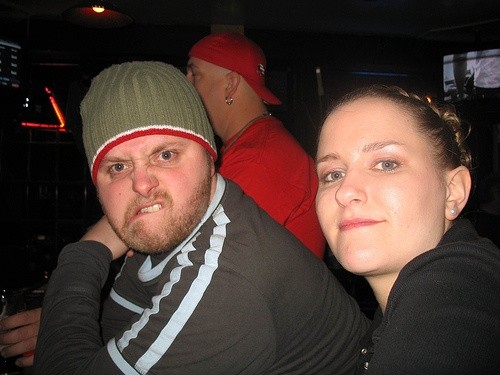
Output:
top-left (0, 30), bottom-right (326, 368)
top-left (315, 84), bottom-right (500, 375)
top-left (29, 60), bottom-right (372, 375)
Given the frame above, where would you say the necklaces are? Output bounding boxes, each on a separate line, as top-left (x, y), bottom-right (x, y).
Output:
top-left (221, 112), bottom-right (272, 159)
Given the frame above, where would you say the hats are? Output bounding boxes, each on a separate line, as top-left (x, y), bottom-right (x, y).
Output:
top-left (188, 32), bottom-right (282, 104)
top-left (79, 61), bottom-right (217, 186)
